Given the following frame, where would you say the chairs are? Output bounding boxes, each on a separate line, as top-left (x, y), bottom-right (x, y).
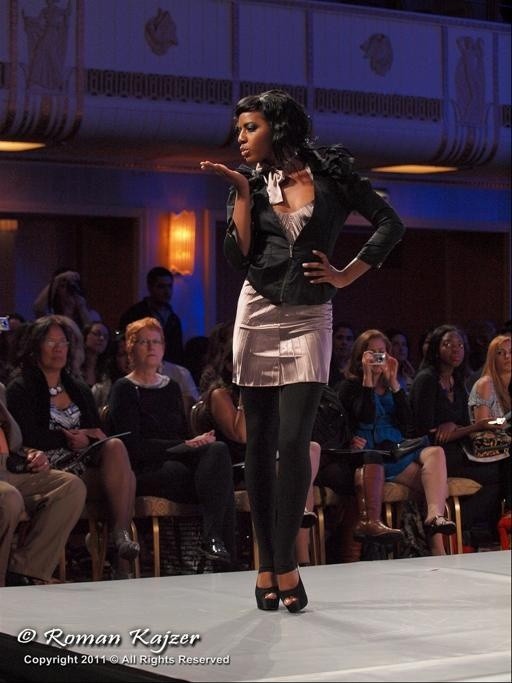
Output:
top-left (0, 477), bottom-right (482, 581)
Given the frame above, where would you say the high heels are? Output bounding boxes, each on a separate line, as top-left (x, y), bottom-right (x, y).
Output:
top-left (106, 528), bottom-right (140, 561)
top-left (426, 516), bottom-right (456, 547)
top-left (109, 559), bottom-right (129, 580)
top-left (497, 517), bottom-right (512, 548)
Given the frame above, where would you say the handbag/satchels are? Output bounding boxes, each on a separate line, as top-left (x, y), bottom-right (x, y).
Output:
top-left (6, 453), bottom-right (31, 473)
top-left (464, 430), bottom-right (511, 457)
top-left (380, 438), bottom-right (425, 462)
top-left (322, 447), bottom-right (390, 464)
top-left (227, 443), bottom-right (246, 490)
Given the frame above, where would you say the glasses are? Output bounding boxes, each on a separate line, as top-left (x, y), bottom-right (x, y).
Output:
top-left (47, 341), bottom-right (70, 349)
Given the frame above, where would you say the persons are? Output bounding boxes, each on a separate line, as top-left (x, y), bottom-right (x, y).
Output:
top-left (199, 89), bottom-right (407, 614)
top-left (0, 267), bottom-right (512, 587)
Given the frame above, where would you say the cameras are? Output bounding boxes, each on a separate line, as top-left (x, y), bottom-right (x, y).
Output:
top-left (368, 351), bottom-right (388, 369)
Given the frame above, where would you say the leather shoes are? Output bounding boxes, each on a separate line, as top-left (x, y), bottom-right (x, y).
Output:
top-left (198, 536), bottom-right (231, 564)
top-left (4, 572), bottom-right (36, 587)
top-left (302, 506), bottom-right (317, 529)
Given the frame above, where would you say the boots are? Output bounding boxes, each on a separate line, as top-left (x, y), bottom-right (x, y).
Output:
top-left (354, 453), bottom-right (402, 544)
top-left (340, 527), bottom-right (362, 562)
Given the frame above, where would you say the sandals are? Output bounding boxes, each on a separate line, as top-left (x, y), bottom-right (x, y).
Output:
top-left (256, 581), bottom-right (280, 610)
top-left (279, 577), bottom-right (308, 613)
